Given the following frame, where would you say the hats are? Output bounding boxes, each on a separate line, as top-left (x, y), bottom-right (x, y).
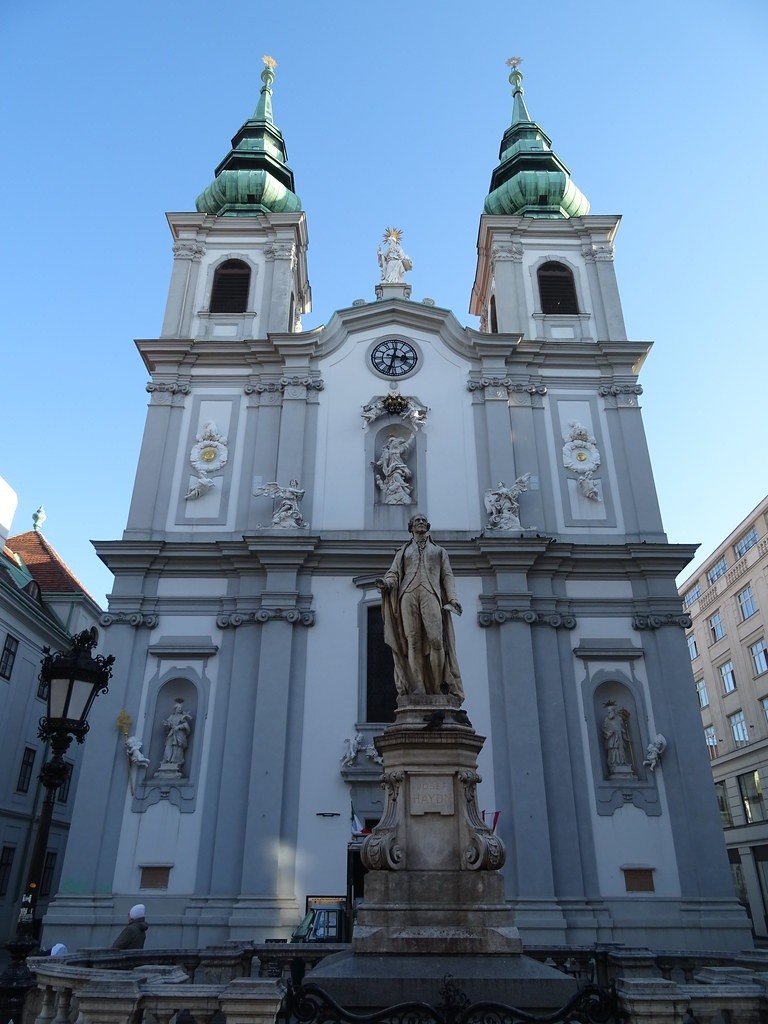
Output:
top-left (130, 904), bottom-right (145, 919)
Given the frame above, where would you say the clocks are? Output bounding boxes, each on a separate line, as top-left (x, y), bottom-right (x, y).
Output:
top-left (371, 340), bottom-right (418, 377)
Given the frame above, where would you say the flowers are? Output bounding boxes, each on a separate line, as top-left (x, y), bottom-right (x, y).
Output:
top-left (117, 709), bottom-right (133, 727)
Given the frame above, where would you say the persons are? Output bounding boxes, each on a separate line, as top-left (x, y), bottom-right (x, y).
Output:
top-left (111, 904), bottom-right (149, 948)
top-left (577, 471), bottom-right (601, 502)
top-left (603, 709), bottom-right (627, 765)
top-left (489, 482), bottom-right (519, 518)
top-left (125, 736), bottom-right (150, 768)
top-left (159, 698), bottom-right (192, 763)
top-left (183, 470), bottom-right (216, 500)
top-left (372, 436), bottom-right (412, 481)
top-left (377, 236), bottom-right (412, 283)
top-left (272, 479), bottom-right (306, 527)
top-left (643, 740), bottom-right (664, 773)
top-left (375, 513), bottom-right (463, 695)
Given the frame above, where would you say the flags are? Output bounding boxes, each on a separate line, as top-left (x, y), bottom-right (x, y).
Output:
top-left (481, 809), bottom-right (499, 834)
top-left (351, 808), bottom-right (371, 834)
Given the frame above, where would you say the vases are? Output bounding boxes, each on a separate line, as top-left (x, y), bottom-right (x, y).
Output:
top-left (122, 725), bottom-right (128, 732)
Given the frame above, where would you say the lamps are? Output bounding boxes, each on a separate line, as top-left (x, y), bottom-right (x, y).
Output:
top-left (381, 390), bottom-right (409, 416)
